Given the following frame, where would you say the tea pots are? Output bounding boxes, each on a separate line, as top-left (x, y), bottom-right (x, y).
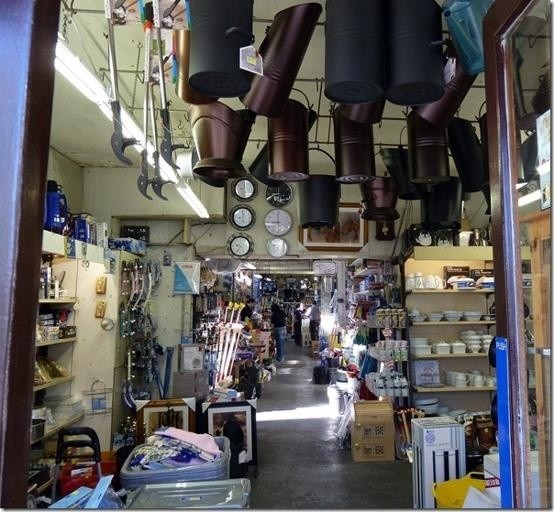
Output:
top-left (407, 271), bottom-right (447, 290)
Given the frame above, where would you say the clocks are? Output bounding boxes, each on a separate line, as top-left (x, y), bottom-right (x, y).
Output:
top-left (223, 170), bottom-right (296, 260)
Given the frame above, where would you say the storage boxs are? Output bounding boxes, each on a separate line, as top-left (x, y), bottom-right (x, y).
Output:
top-left (125, 476), bottom-right (256, 509)
top-left (171, 385), bottom-right (209, 403)
top-left (170, 370), bottom-right (210, 386)
top-left (120, 434), bottom-right (234, 488)
top-left (348, 435), bottom-right (399, 463)
top-left (347, 399), bottom-right (397, 441)
top-left (480, 445), bottom-right (543, 509)
top-left (107, 236), bottom-right (147, 256)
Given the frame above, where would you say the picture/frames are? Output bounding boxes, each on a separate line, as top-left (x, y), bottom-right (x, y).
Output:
top-left (200, 398), bottom-right (260, 471)
top-left (297, 199), bottom-right (371, 254)
top-left (133, 395), bottom-right (198, 442)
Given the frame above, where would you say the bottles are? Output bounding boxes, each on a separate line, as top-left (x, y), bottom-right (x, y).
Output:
top-left (371, 304), bottom-right (409, 398)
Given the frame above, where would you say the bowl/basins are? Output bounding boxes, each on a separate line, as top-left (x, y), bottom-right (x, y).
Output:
top-left (416, 398), bottom-right (466, 416)
top-left (409, 304), bottom-right (493, 355)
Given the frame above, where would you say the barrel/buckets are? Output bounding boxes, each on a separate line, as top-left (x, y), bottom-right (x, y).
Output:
top-left (515, 123), bottom-right (526, 180)
top-left (513, 47), bottom-right (528, 118)
top-left (313, 366), bottom-right (329, 384)
top-left (530, 71), bottom-right (550, 115)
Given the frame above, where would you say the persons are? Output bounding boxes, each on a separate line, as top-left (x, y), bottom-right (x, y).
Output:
top-left (271, 304), bottom-right (288, 362)
top-left (309, 301), bottom-right (320, 348)
top-left (293, 304), bottom-right (306, 345)
top-left (241, 297), bottom-right (256, 332)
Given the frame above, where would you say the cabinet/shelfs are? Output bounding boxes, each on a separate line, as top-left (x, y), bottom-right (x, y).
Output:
top-left (396, 242), bottom-right (537, 432)
top-left (27, 222), bottom-right (116, 509)
top-left (517, 200), bottom-right (552, 509)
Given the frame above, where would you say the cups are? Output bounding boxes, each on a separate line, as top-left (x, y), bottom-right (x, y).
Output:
top-left (43, 177), bottom-right (66, 233)
top-left (443, 366), bottom-right (495, 388)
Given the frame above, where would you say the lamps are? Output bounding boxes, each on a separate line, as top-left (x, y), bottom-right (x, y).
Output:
top-left (52, 0), bottom-right (211, 221)
